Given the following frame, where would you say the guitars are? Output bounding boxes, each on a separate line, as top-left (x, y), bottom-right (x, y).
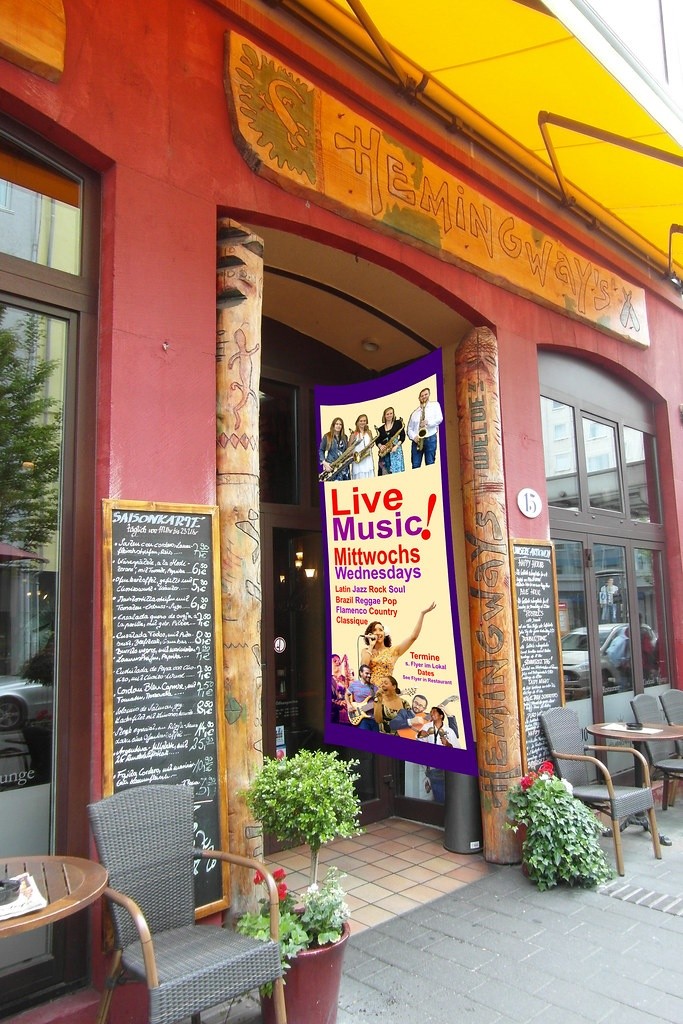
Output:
top-left (347, 687), bottom-right (417, 726)
top-left (397, 696), bottom-right (459, 740)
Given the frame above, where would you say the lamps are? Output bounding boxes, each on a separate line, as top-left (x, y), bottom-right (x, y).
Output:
top-left (279, 552), bottom-right (319, 584)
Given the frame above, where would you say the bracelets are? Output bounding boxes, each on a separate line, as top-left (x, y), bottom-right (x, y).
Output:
top-left (366, 650), bottom-right (372, 655)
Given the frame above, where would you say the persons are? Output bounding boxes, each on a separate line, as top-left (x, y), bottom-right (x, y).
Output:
top-left (360, 601), bottom-right (437, 690)
top-left (623, 612), bottom-right (653, 676)
top-left (318, 417), bottom-right (351, 481)
top-left (375, 407), bottom-right (406, 476)
top-left (348, 414), bottom-right (375, 480)
top-left (407, 388), bottom-right (443, 469)
top-left (601, 578), bottom-right (618, 620)
top-left (332, 654), bottom-right (460, 749)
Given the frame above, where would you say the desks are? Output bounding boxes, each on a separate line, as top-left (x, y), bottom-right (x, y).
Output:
top-left (4, 738), bottom-right (26, 744)
top-left (584, 723), bottom-right (682, 847)
top-left (0, 857), bottom-right (109, 939)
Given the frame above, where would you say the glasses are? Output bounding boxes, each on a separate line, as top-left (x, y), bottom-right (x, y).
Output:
top-left (413, 701), bottom-right (426, 709)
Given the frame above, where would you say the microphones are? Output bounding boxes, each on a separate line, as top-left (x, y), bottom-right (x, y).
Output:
top-left (372, 689), bottom-right (384, 699)
top-left (361, 634), bottom-right (376, 640)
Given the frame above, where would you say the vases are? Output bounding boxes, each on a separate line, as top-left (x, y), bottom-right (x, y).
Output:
top-left (513, 820), bottom-right (530, 879)
top-left (22, 726), bottom-right (51, 785)
top-left (258, 907), bottom-right (352, 1024)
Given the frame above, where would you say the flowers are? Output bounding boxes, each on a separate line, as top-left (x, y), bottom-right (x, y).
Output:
top-left (233, 750), bottom-right (367, 1000)
top-left (27, 708), bottom-right (51, 731)
top-left (501, 762), bottom-right (618, 892)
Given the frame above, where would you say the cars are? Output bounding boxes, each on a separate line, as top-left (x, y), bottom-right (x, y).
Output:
top-left (0, 675), bottom-right (54, 730)
top-left (561, 623), bottom-right (658, 695)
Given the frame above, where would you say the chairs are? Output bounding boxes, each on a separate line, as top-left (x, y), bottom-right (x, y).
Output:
top-left (0, 748), bottom-right (28, 785)
top-left (87, 785), bottom-right (289, 1024)
top-left (538, 706), bottom-right (663, 876)
top-left (631, 689), bottom-right (683, 810)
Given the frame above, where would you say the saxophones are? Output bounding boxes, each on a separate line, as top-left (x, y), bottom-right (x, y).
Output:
top-left (339, 655), bottom-right (351, 689)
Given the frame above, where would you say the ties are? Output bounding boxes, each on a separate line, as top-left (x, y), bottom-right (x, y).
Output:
top-left (419, 407), bottom-right (424, 450)
